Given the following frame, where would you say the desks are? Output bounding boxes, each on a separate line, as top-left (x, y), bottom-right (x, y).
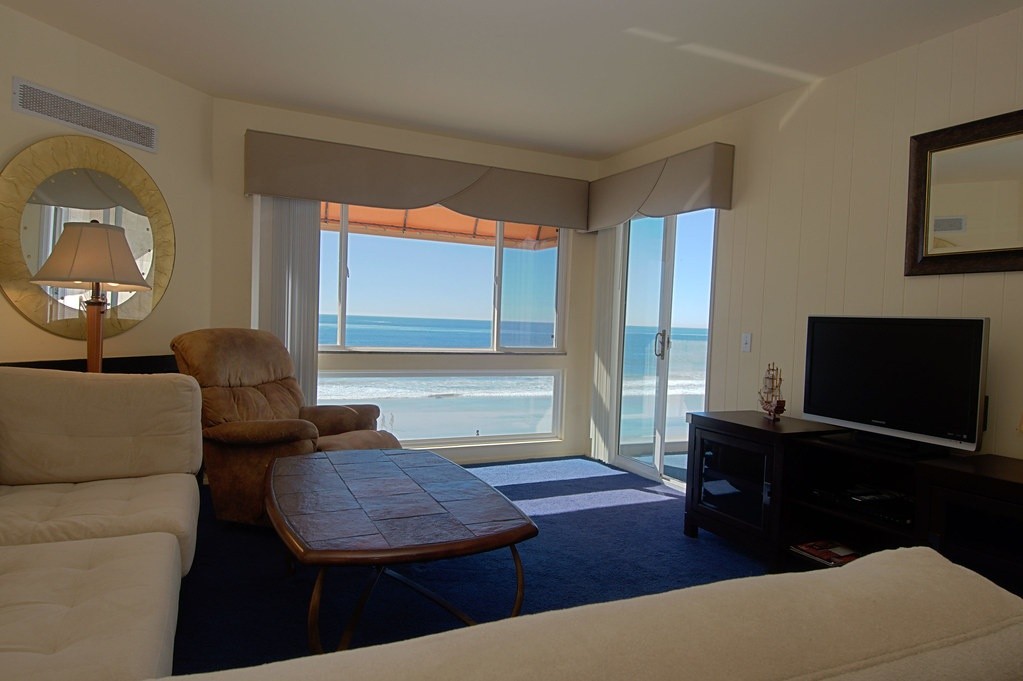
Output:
top-left (262, 448), bottom-right (539, 652)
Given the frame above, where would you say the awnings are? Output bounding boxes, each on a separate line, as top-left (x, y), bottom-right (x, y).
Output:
top-left (321, 203), bottom-right (558, 250)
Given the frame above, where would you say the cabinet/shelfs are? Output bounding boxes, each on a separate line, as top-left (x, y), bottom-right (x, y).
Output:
top-left (684, 409), bottom-right (1023, 601)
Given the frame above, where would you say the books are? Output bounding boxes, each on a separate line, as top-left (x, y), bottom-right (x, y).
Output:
top-left (790, 538), bottom-right (864, 566)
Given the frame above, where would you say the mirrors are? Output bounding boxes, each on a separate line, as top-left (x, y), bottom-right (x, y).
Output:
top-left (0, 135), bottom-right (176, 339)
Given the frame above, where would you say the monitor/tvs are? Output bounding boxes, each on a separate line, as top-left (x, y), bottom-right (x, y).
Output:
top-left (802, 316), bottom-right (990, 459)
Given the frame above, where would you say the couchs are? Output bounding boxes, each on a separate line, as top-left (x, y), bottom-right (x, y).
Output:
top-left (158, 547), bottom-right (1023, 681)
top-left (170, 329), bottom-right (403, 520)
top-left (0, 367), bottom-right (203, 681)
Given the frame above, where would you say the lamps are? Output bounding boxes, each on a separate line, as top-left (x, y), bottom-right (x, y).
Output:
top-left (30, 220), bottom-right (152, 374)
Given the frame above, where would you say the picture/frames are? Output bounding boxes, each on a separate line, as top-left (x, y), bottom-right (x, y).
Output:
top-left (903, 108), bottom-right (1023, 276)
